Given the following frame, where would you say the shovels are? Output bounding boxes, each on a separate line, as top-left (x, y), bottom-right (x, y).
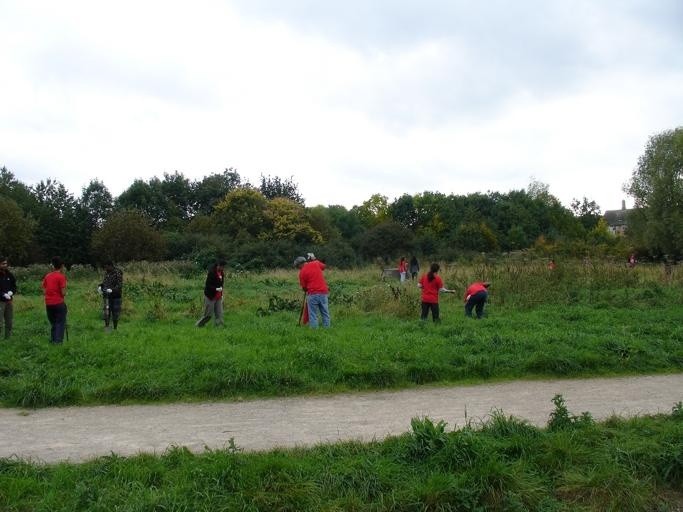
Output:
top-left (103, 287), bottom-right (112, 334)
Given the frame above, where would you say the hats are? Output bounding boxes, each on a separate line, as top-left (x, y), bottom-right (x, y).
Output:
top-left (292, 256), bottom-right (306, 268)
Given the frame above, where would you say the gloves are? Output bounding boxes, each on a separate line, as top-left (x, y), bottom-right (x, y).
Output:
top-left (216, 286), bottom-right (223, 292)
top-left (97, 286), bottom-right (102, 293)
top-left (106, 288), bottom-right (112, 293)
top-left (3, 291), bottom-right (14, 300)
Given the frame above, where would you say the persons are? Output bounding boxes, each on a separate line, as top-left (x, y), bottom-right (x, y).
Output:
top-left (194, 258), bottom-right (227, 329)
top-left (0, 255), bottom-right (18, 340)
top-left (462, 281), bottom-right (491, 320)
top-left (39, 255), bottom-right (67, 344)
top-left (407, 255), bottom-right (420, 280)
top-left (96, 259), bottom-right (124, 330)
top-left (626, 253), bottom-right (638, 268)
top-left (397, 256), bottom-right (407, 281)
top-left (415, 261), bottom-right (444, 326)
top-left (546, 258), bottom-right (556, 269)
top-left (292, 252), bottom-right (331, 330)
